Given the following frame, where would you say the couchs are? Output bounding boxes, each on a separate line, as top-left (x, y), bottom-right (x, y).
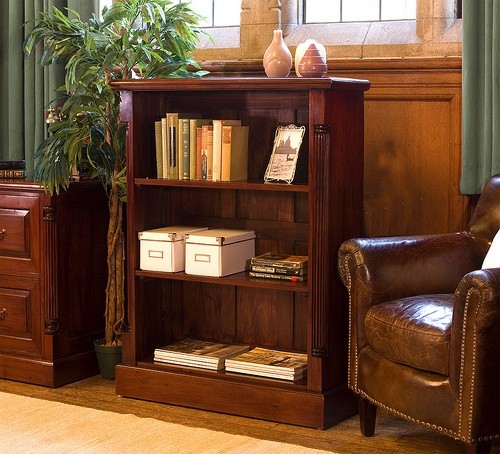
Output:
top-left (338, 174), bottom-right (499, 454)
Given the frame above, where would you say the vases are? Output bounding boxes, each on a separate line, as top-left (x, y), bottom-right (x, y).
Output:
top-left (263, 29), bottom-right (292, 78)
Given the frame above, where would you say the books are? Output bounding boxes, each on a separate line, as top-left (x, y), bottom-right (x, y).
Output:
top-left (154, 337), bottom-right (250, 370)
top-left (225, 346), bottom-right (309, 382)
top-left (155, 112), bottom-right (249, 182)
top-left (249, 251), bottom-right (308, 282)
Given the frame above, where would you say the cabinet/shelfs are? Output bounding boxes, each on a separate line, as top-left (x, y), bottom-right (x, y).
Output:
top-left (0, 179), bottom-right (110, 388)
top-left (107, 76), bottom-right (370, 431)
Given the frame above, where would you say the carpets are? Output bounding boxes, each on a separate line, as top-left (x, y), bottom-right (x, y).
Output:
top-left (0, 391), bottom-right (336, 454)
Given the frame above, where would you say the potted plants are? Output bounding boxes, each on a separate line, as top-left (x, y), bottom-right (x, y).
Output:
top-left (19, 0), bottom-right (215, 383)
